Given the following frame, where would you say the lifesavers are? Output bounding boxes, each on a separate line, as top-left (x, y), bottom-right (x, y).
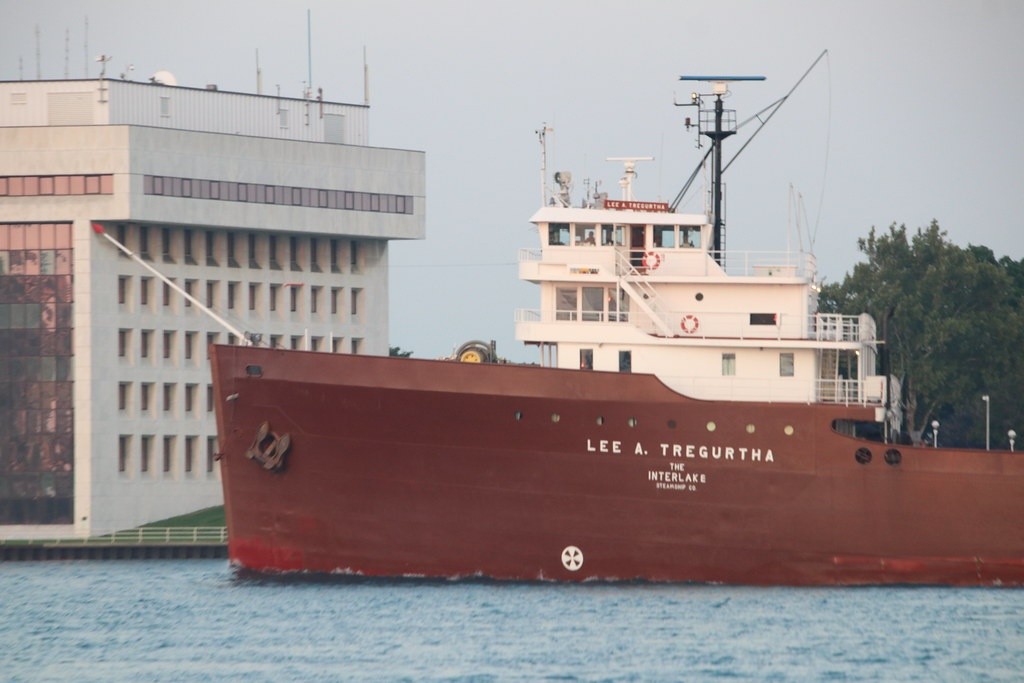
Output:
top-left (681, 313), bottom-right (698, 334)
top-left (641, 252), bottom-right (661, 272)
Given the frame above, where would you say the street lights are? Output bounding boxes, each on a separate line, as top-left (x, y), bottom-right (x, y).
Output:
top-left (931, 419), bottom-right (939, 448)
top-left (981, 395), bottom-right (990, 449)
top-left (1007, 428), bottom-right (1017, 453)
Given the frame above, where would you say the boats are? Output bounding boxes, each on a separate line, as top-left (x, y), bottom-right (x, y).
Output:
top-left (207, 75), bottom-right (1024, 593)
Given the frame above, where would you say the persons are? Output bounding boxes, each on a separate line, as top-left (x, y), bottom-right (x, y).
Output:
top-left (585, 231), bottom-right (595, 245)
